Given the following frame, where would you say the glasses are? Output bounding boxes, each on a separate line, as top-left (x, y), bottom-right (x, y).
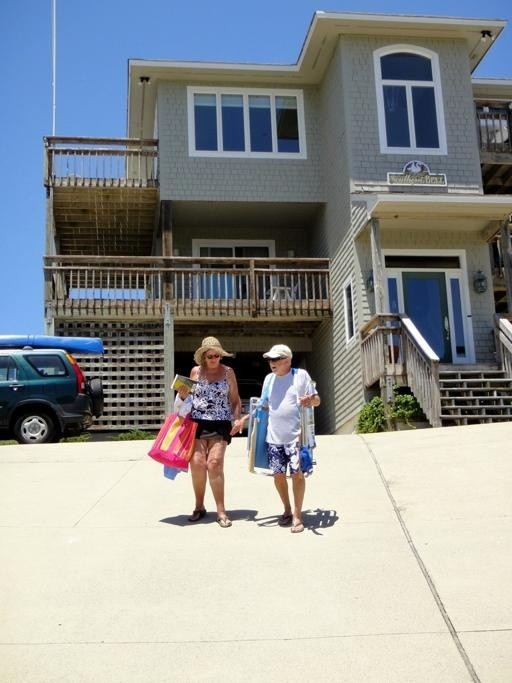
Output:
top-left (206, 354), bottom-right (220, 360)
top-left (266, 358), bottom-right (280, 363)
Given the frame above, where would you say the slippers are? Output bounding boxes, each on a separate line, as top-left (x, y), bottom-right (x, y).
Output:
top-left (291, 520), bottom-right (304, 533)
top-left (188, 509), bottom-right (206, 522)
top-left (216, 515), bottom-right (232, 528)
top-left (277, 513), bottom-right (292, 525)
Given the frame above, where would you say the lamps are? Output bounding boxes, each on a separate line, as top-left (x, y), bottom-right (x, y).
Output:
top-left (476, 267), bottom-right (487, 296)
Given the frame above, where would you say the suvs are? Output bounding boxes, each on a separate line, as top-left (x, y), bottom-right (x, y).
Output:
top-left (1, 345), bottom-right (106, 444)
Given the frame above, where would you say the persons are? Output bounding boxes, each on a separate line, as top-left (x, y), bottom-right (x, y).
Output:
top-left (176, 336), bottom-right (243, 528)
top-left (230, 343), bottom-right (322, 534)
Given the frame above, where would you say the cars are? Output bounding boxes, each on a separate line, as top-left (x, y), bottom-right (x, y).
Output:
top-left (232, 377), bottom-right (262, 432)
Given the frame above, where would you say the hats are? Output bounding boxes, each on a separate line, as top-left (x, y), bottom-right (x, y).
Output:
top-left (263, 344), bottom-right (292, 359)
top-left (193, 336), bottom-right (233, 365)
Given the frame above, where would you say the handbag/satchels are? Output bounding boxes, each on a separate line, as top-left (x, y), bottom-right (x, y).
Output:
top-left (148, 414), bottom-right (198, 468)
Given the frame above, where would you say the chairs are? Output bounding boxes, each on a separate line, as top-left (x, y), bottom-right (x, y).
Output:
top-left (264, 278), bottom-right (299, 299)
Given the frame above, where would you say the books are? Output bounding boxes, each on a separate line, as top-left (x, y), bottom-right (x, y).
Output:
top-left (171, 373), bottom-right (199, 395)
